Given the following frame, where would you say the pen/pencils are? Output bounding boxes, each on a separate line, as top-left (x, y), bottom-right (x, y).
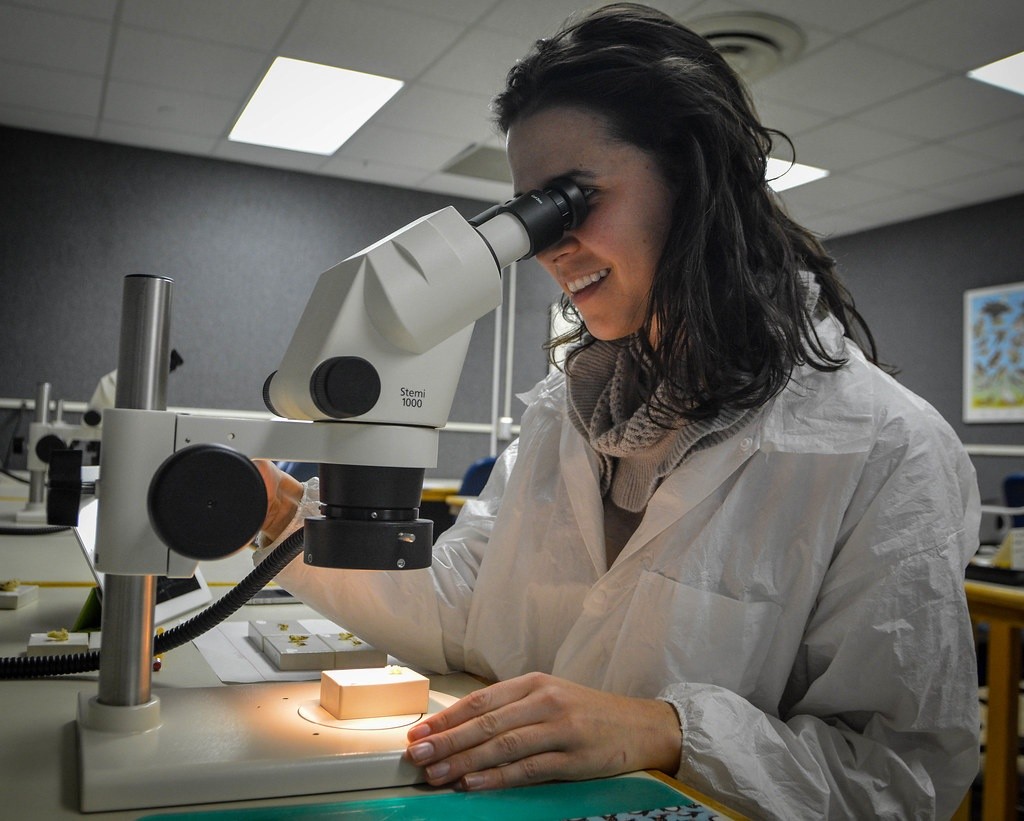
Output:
top-left (152, 625), bottom-right (165, 672)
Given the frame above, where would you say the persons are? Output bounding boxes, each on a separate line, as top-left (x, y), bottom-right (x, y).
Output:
top-left (86, 368), bottom-right (118, 413)
top-left (252, 2), bottom-right (981, 821)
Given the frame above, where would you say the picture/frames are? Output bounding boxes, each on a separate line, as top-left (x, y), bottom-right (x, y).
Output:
top-left (962, 282), bottom-right (1024, 425)
